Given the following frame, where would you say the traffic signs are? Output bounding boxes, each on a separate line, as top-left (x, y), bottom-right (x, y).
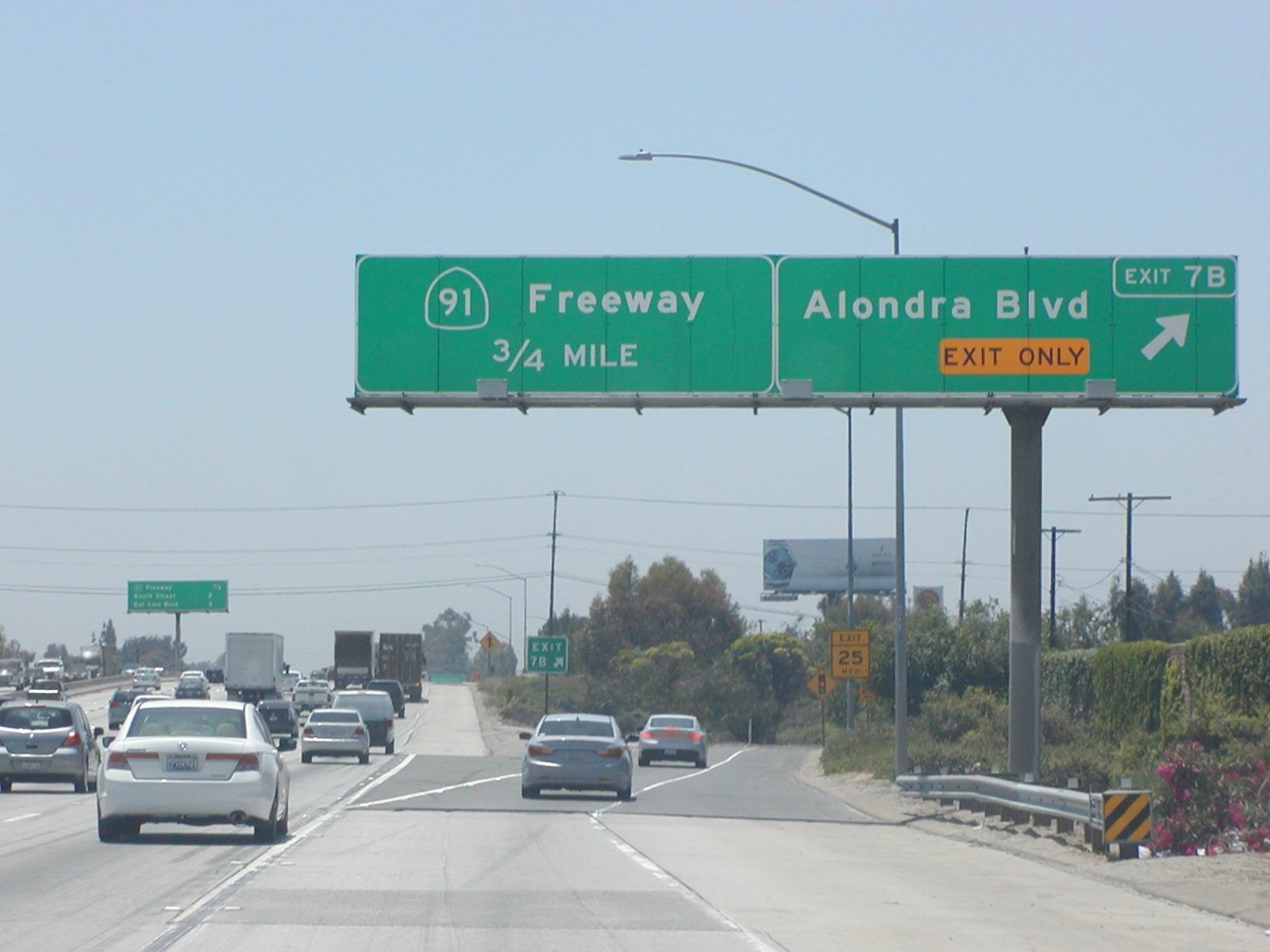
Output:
top-left (526, 635), bottom-right (568, 674)
top-left (772, 255), bottom-right (1240, 400)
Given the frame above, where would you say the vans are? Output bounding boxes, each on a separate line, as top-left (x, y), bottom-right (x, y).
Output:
top-left (332, 689), bottom-right (394, 755)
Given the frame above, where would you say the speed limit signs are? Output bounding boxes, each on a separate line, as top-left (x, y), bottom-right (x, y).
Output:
top-left (831, 630), bottom-right (871, 680)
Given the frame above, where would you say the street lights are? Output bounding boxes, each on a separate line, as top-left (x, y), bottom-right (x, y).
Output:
top-left (617, 147), bottom-right (906, 783)
top-left (467, 584), bottom-right (512, 647)
top-left (475, 564), bottom-right (527, 676)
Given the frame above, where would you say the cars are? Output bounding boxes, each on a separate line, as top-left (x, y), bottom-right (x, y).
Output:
top-left (0, 656), bottom-right (428, 731)
top-left (96, 699), bottom-right (290, 844)
top-left (637, 713), bottom-right (710, 769)
top-left (299, 708), bottom-right (372, 764)
top-left (0, 701), bottom-right (104, 794)
top-left (519, 713), bottom-right (639, 801)
top-left (257, 698), bottom-right (300, 751)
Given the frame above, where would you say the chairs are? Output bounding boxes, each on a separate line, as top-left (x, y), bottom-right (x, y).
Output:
top-left (143, 723), bottom-right (162, 735)
top-left (4, 715), bottom-right (29, 727)
top-left (48, 716), bottom-right (65, 726)
top-left (216, 723), bottom-right (240, 737)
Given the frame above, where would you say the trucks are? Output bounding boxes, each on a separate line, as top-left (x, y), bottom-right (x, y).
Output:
top-left (223, 632), bottom-right (290, 704)
top-left (378, 633), bottom-right (426, 702)
top-left (335, 629), bottom-right (373, 691)
